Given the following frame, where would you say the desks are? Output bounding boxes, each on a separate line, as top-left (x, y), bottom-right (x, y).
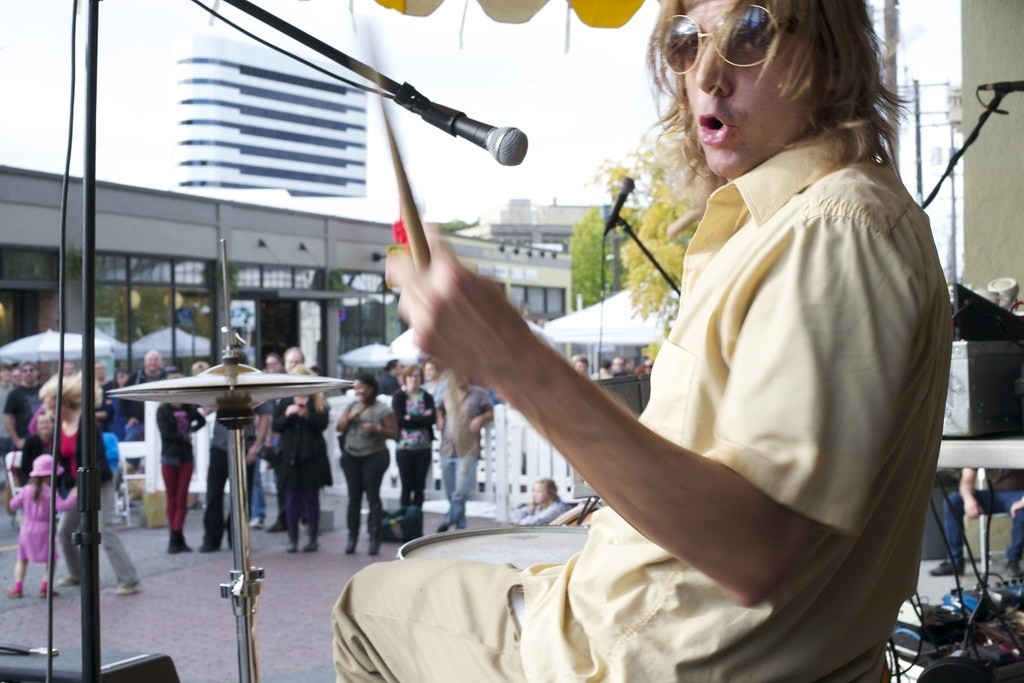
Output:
top-left (931, 437), bottom-right (1024, 469)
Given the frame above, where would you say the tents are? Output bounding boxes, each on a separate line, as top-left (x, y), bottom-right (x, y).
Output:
top-left (339, 330), bottom-right (428, 370)
top-left (544, 281), bottom-right (679, 369)
top-left (97, 326), bottom-right (209, 361)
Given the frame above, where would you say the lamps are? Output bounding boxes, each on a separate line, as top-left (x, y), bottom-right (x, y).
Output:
top-left (551, 250), bottom-right (557, 258)
top-left (512, 240), bottom-right (521, 255)
top-left (539, 248), bottom-right (545, 258)
top-left (373, 253), bottom-right (387, 262)
top-left (499, 239), bottom-right (507, 252)
top-left (257, 238), bottom-right (281, 273)
top-left (526, 242), bottom-right (532, 257)
top-left (297, 243), bottom-right (327, 278)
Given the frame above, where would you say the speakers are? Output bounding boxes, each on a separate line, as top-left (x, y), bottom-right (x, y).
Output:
top-left (0, 642), bottom-right (180, 683)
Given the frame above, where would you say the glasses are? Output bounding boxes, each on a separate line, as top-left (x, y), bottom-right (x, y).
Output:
top-left (663, 4), bottom-right (786, 74)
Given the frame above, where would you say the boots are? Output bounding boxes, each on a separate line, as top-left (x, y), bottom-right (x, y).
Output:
top-left (169, 532), bottom-right (191, 553)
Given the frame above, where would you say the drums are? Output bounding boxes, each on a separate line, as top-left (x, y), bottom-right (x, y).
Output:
top-left (395, 526), bottom-right (591, 570)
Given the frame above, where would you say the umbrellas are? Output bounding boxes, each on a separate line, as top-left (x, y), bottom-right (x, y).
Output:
top-left (0, 328), bottom-right (111, 364)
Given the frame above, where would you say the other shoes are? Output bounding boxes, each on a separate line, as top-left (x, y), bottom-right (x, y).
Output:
top-left (41, 590), bottom-right (58, 597)
top-left (1006, 564), bottom-right (1023, 577)
top-left (370, 535), bottom-right (379, 554)
top-left (346, 532), bottom-right (358, 552)
top-left (287, 544), bottom-right (296, 551)
top-left (930, 562), bottom-right (965, 576)
top-left (437, 526), bottom-right (447, 532)
top-left (199, 535), bottom-right (221, 552)
top-left (57, 576), bottom-right (79, 585)
top-left (249, 518), bottom-right (264, 528)
top-left (304, 544), bottom-right (317, 551)
top-left (7, 589), bottom-right (21, 597)
top-left (267, 520), bottom-right (287, 532)
top-left (117, 582), bottom-right (141, 593)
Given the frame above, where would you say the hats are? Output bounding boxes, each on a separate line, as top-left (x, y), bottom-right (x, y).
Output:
top-left (29, 454), bottom-right (64, 477)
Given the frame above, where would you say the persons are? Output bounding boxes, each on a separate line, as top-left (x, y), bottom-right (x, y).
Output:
top-left (573, 355), bottom-right (651, 382)
top-left (0, 347), bottom-right (501, 600)
top-left (511, 479), bottom-right (568, 527)
top-left (931, 467), bottom-right (1024, 578)
top-left (331, 1), bottom-right (952, 683)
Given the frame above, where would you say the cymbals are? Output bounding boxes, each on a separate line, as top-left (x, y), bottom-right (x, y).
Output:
top-left (104, 363), bottom-right (356, 409)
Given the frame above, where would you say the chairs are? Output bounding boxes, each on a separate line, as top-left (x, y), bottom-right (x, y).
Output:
top-left (977, 467), bottom-right (1012, 575)
top-left (5, 451), bottom-right (24, 531)
top-left (117, 440), bottom-right (147, 529)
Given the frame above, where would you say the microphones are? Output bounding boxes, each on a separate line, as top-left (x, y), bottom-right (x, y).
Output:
top-left (977, 81), bottom-right (1024, 92)
top-left (604, 178), bottom-right (635, 236)
top-left (394, 96), bottom-right (528, 166)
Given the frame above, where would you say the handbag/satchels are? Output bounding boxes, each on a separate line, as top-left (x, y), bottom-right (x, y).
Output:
top-left (383, 504), bottom-right (422, 541)
top-left (338, 435), bottom-right (345, 451)
